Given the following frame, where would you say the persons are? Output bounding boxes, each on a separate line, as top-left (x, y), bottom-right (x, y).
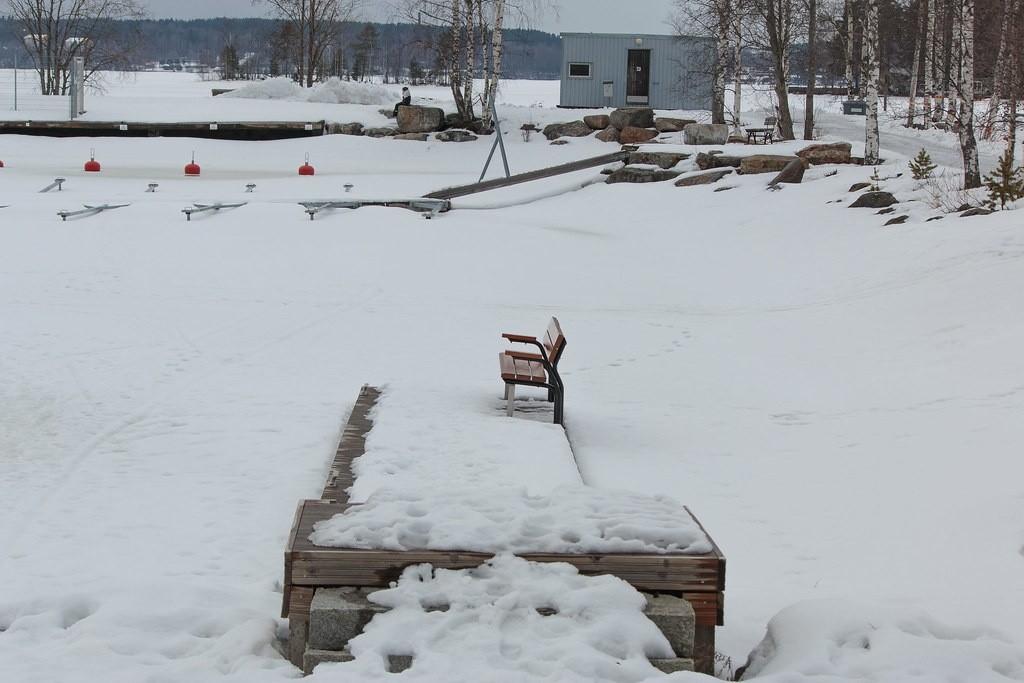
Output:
top-left (392, 87), bottom-right (410, 117)
